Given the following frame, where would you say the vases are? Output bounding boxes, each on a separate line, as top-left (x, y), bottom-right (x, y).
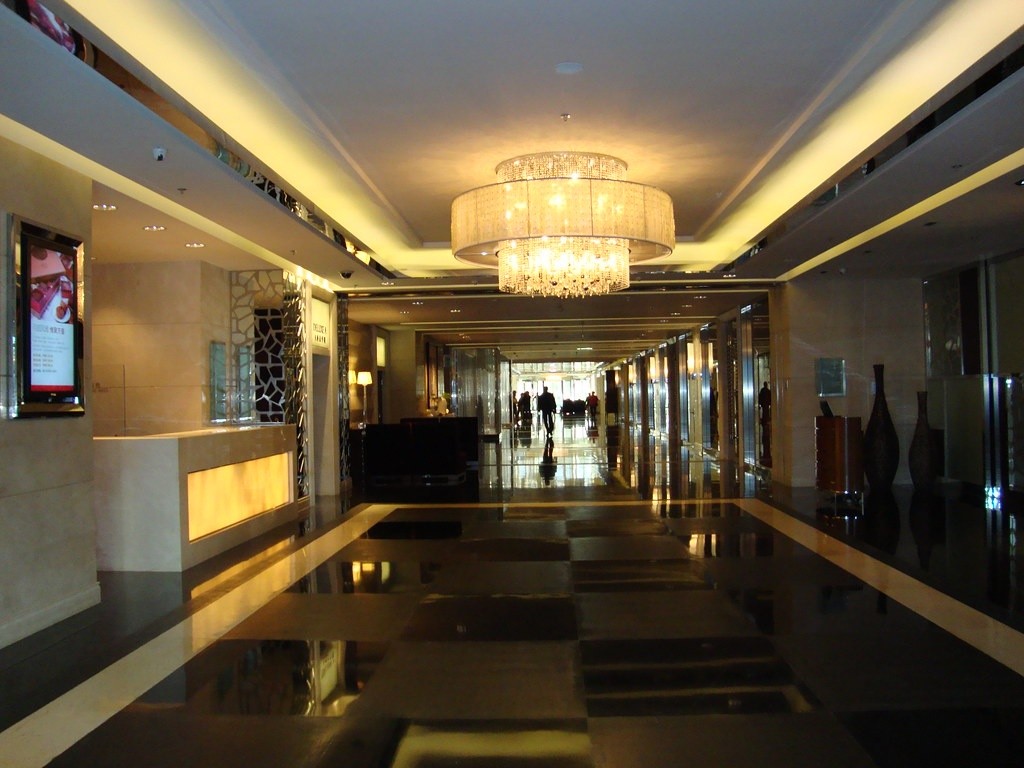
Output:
top-left (861, 364), bottom-right (899, 490)
top-left (908, 392), bottom-right (939, 491)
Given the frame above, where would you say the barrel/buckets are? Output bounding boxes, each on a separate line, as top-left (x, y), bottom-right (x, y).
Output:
top-left (813, 414), bottom-right (864, 496)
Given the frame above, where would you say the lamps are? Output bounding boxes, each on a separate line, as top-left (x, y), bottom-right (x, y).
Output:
top-left (450, 152), bottom-right (676, 298)
top-left (357, 372), bottom-right (373, 424)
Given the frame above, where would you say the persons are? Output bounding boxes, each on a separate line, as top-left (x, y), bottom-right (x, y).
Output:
top-left (586, 391), bottom-right (598, 418)
top-left (512, 390), bottom-right (539, 426)
top-left (537, 386), bottom-right (556, 436)
top-left (540, 437), bottom-right (558, 486)
top-left (758, 381), bottom-right (771, 421)
